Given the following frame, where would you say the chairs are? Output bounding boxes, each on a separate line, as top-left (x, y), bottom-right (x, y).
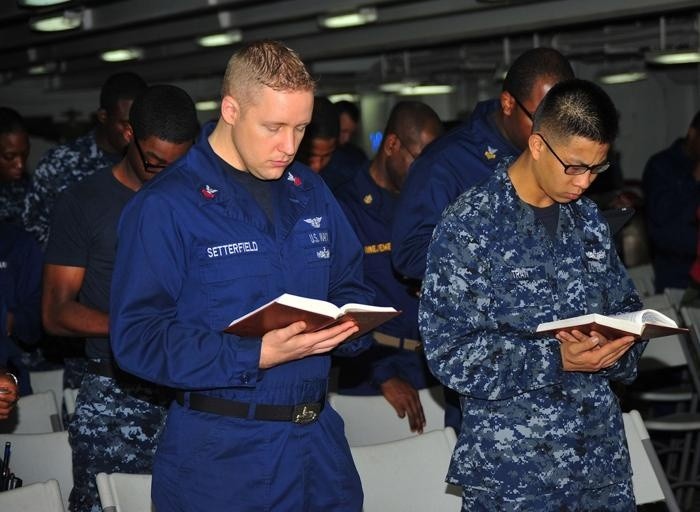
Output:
top-left (619, 266), bottom-right (699, 512)
top-left (1, 370), bottom-right (677, 512)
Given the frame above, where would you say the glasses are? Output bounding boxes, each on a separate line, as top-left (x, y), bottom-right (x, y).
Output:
top-left (133, 137), bottom-right (170, 175)
top-left (538, 132), bottom-right (610, 176)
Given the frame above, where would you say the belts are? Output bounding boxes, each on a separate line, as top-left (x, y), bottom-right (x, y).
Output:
top-left (178, 390), bottom-right (328, 424)
top-left (370, 329), bottom-right (426, 356)
top-left (89, 360), bottom-right (172, 406)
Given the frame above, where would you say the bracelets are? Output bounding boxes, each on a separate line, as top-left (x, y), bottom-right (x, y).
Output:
top-left (6, 372), bottom-right (18, 385)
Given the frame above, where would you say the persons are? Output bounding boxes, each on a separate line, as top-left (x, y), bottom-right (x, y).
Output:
top-left (639, 112), bottom-right (699, 294)
top-left (1, 216), bottom-right (31, 433)
top-left (335, 100), bottom-right (444, 434)
top-left (20, 72), bottom-right (149, 372)
top-left (1, 107), bottom-right (33, 216)
top-left (584, 110), bottom-right (640, 266)
top-left (295, 94), bottom-right (354, 196)
top-left (108, 38), bottom-right (377, 510)
top-left (41, 84), bottom-right (198, 509)
top-left (328, 101), bottom-right (369, 181)
top-left (390, 47), bottom-right (576, 444)
top-left (418, 80), bottom-right (649, 512)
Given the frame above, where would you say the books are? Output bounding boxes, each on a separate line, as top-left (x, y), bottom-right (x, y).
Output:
top-left (222, 293), bottom-right (403, 348)
top-left (536, 309), bottom-right (693, 346)
